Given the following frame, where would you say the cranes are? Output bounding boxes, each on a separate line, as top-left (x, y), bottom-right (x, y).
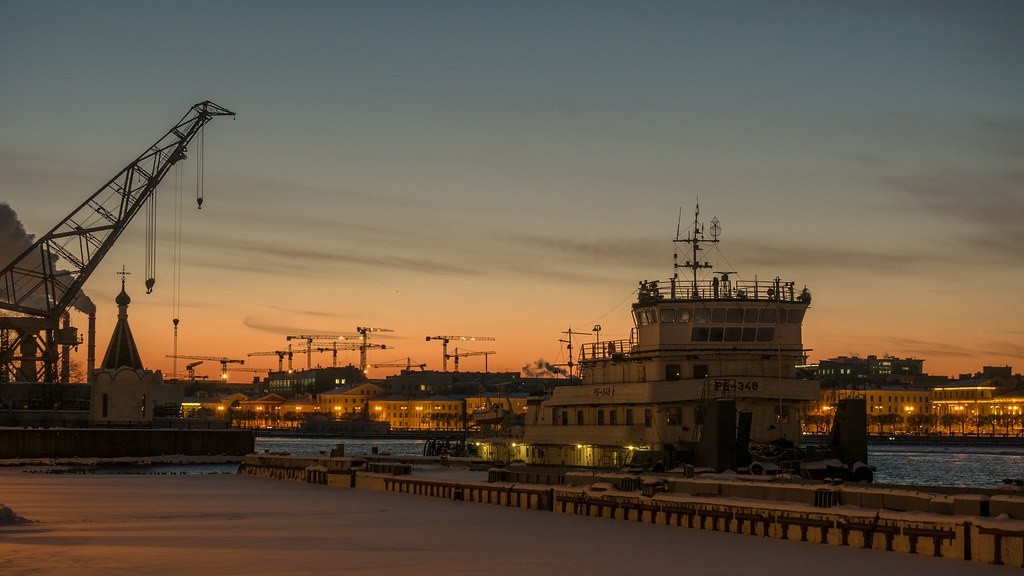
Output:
top-left (552, 324), bottom-right (592, 382)
top-left (166, 326), bottom-right (396, 381)
top-left (366, 357), bottom-right (427, 371)
top-left (446, 347), bottom-right (497, 371)
top-left (426, 335), bottom-right (496, 372)
